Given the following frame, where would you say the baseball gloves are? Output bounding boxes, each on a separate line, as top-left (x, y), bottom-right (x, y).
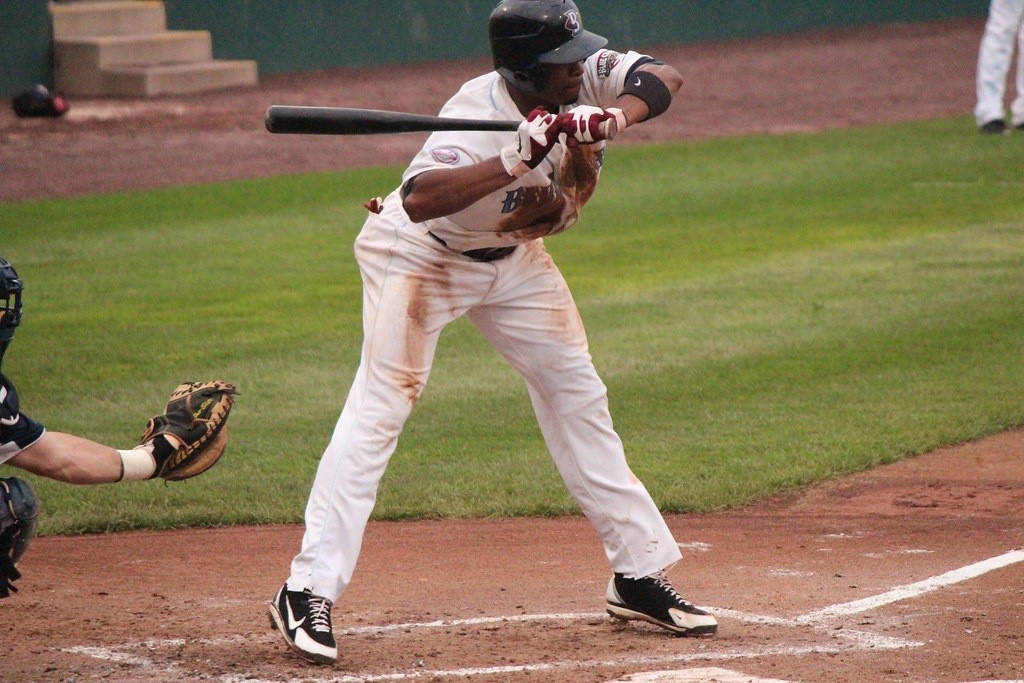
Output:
top-left (138, 377), bottom-right (236, 487)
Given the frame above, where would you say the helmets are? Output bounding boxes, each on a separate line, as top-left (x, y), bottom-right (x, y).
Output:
top-left (488, 0), bottom-right (608, 91)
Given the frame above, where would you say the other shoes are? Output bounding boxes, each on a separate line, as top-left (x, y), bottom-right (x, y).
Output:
top-left (981, 120), bottom-right (1011, 137)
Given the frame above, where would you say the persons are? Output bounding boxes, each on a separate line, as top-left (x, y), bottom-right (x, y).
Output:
top-left (975, 0), bottom-right (1024, 133)
top-left (0, 258), bottom-right (237, 600)
top-left (269, 0), bottom-right (717, 663)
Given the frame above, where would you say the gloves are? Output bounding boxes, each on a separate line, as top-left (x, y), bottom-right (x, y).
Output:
top-left (363, 193), bottom-right (382, 214)
top-left (500, 105), bottom-right (563, 177)
top-left (564, 105), bottom-right (628, 148)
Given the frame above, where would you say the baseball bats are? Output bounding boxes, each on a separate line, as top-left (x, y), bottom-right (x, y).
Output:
top-left (265, 100), bottom-right (619, 151)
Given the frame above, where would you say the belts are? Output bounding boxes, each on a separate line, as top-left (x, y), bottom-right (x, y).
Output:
top-left (429, 232), bottom-right (519, 263)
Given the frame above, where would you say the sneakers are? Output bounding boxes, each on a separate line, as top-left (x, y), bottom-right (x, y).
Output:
top-left (606, 572), bottom-right (718, 637)
top-left (267, 582), bottom-right (338, 664)
